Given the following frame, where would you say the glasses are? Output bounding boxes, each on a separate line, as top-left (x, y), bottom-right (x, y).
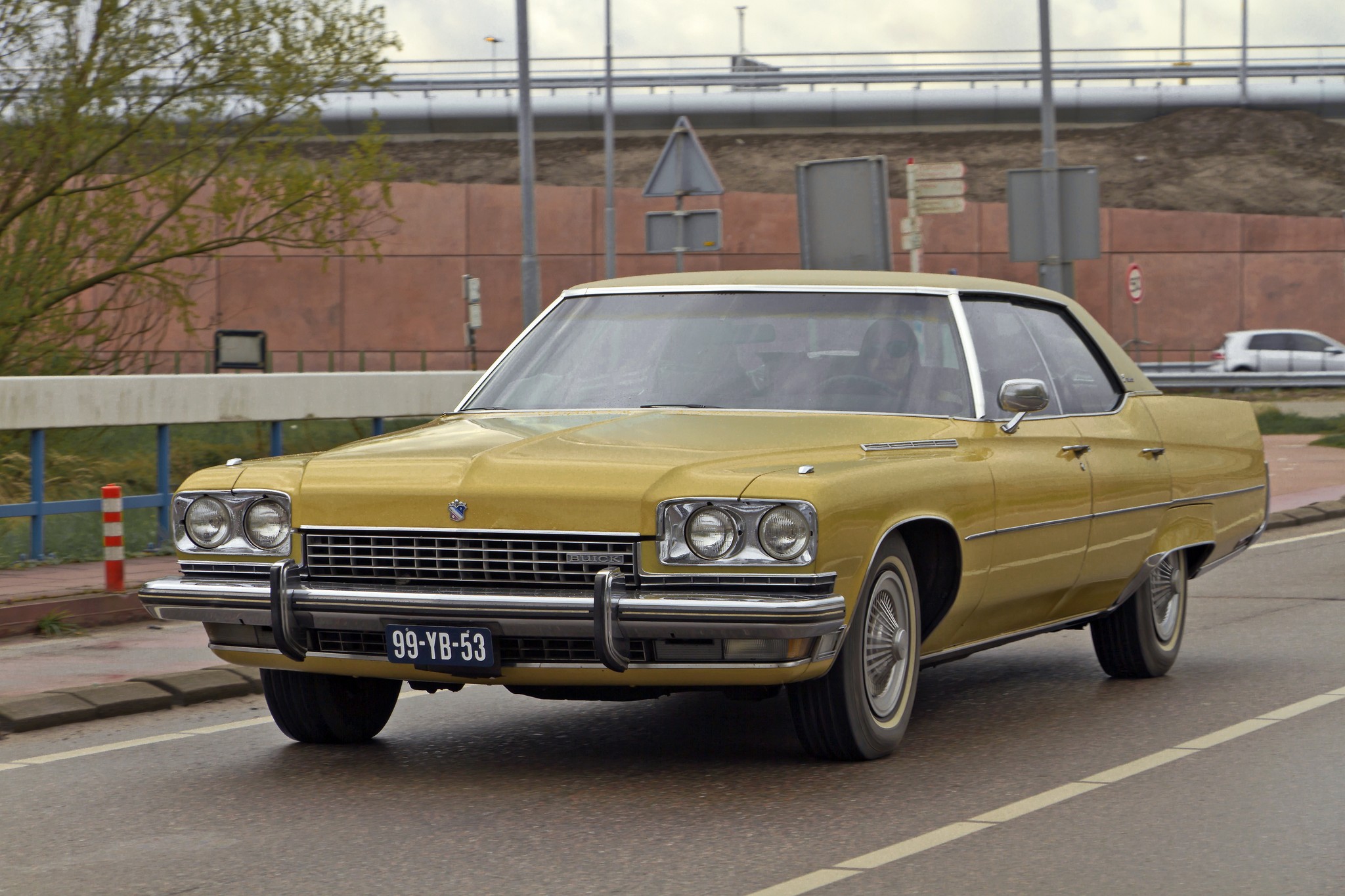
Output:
top-left (868, 340), bottom-right (912, 361)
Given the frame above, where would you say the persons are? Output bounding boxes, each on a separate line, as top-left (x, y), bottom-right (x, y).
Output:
top-left (821, 316), bottom-right (950, 417)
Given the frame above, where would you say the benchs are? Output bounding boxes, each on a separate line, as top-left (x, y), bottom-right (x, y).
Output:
top-left (760, 346), bottom-right (960, 398)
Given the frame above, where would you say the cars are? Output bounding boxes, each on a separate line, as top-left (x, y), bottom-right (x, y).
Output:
top-left (141, 271), bottom-right (1272, 769)
top-left (1210, 327), bottom-right (1345, 373)
